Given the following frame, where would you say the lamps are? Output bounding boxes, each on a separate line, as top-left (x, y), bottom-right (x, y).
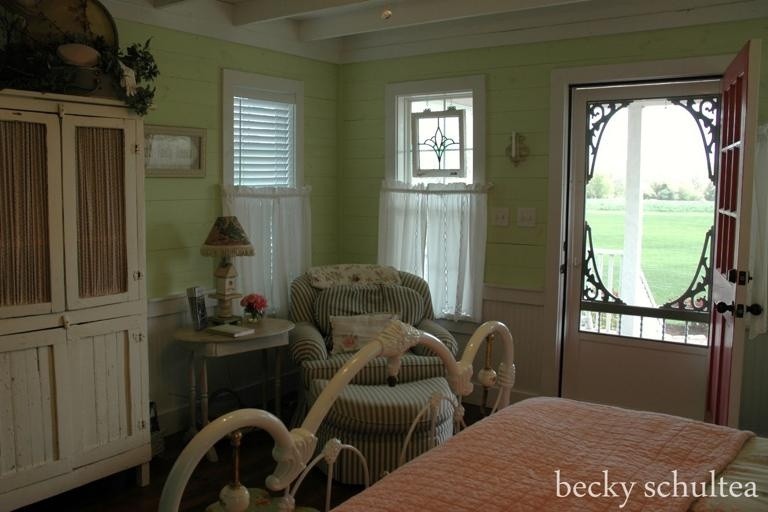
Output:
top-left (287, 264), bottom-right (459, 484)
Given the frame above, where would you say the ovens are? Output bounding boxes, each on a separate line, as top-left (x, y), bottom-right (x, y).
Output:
top-left (157, 319), bottom-right (768, 512)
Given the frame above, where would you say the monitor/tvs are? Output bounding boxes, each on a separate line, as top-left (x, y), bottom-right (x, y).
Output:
top-left (207, 323), bottom-right (257, 338)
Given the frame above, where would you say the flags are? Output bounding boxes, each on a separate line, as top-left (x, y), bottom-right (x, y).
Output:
top-left (247, 311), bottom-right (262, 325)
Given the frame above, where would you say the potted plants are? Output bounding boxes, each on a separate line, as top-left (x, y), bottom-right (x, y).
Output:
top-left (145, 124), bottom-right (208, 179)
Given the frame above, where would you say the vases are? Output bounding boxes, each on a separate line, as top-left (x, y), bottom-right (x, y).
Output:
top-left (242, 292), bottom-right (269, 316)
top-left (0, 1), bottom-right (159, 118)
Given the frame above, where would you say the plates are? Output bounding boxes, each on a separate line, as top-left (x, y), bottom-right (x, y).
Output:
top-left (0, 90), bottom-right (152, 511)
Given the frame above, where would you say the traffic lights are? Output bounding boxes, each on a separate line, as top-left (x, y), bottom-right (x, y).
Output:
top-left (328, 311), bottom-right (405, 353)
top-left (313, 284), bottom-right (426, 335)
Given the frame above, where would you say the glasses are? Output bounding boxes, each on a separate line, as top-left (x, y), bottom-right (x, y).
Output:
top-left (200, 215), bottom-right (256, 324)
